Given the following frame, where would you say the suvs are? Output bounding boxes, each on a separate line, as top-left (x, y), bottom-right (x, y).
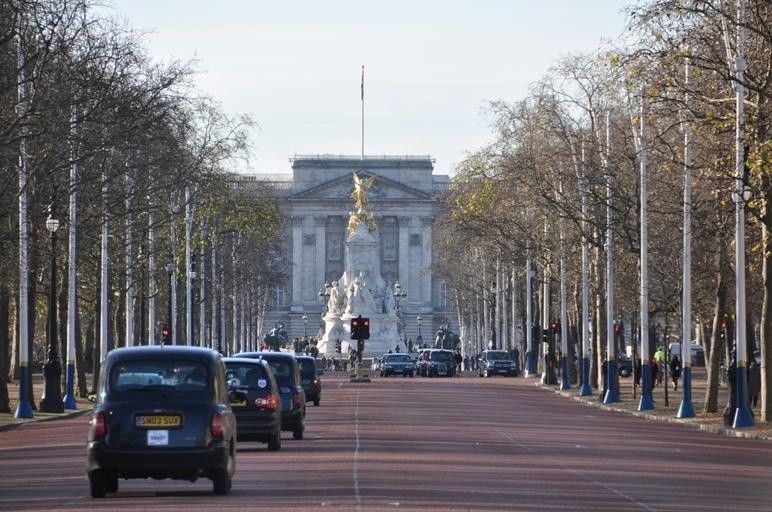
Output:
top-left (85, 343), bottom-right (321, 500)
top-left (477, 348), bottom-right (518, 378)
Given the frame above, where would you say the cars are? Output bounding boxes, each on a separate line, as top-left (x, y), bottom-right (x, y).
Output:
top-left (616, 349), bottom-right (632, 377)
top-left (376, 347), bottom-right (456, 376)
top-left (668, 340), bottom-right (704, 367)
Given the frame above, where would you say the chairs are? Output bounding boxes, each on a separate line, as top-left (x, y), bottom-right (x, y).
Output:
top-left (118, 368), bottom-right (205, 392)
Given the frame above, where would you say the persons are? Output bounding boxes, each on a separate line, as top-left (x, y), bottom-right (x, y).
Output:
top-left (634, 354), bottom-right (642, 387)
top-left (258, 323), bottom-right (416, 370)
top-left (348, 177), bottom-right (379, 238)
top-left (649, 347), bottom-right (681, 392)
top-left (323, 269), bottom-right (407, 315)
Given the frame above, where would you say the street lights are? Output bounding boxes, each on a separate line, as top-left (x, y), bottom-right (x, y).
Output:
top-left (303, 312), bottom-right (308, 337)
top-left (39, 192), bottom-right (68, 413)
top-left (529, 263), bottom-right (537, 355)
top-left (394, 280), bottom-right (406, 344)
top-left (167, 254), bottom-right (175, 347)
top-left (723, 182), bottom-right (755, 429)
top-left (416, 314), bottom-right (424, 348)
top-left (136, 242), bottom-right (147, 347)
top-left (488, 281), bottom-right (496, 349)
top-left (189, 255), bottom-right (196, 347)
top-left (318, 282), bottom-right (332, 310)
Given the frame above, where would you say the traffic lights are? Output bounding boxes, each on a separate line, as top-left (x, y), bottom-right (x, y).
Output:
top-left (550, 322), bottom-right (557, 334)
top-left (335, 343), bottom-right (340, 353)
top-left (350, 318), bottom-right (360, 340)
top-left (613, 323), bottom-right (621, 335)
top-left (542, 329), bottom-right (549, 341)
top-left (162, 327), bottom-right (169, 344)
top-left (360, 318), bottom-right (370, 339)
